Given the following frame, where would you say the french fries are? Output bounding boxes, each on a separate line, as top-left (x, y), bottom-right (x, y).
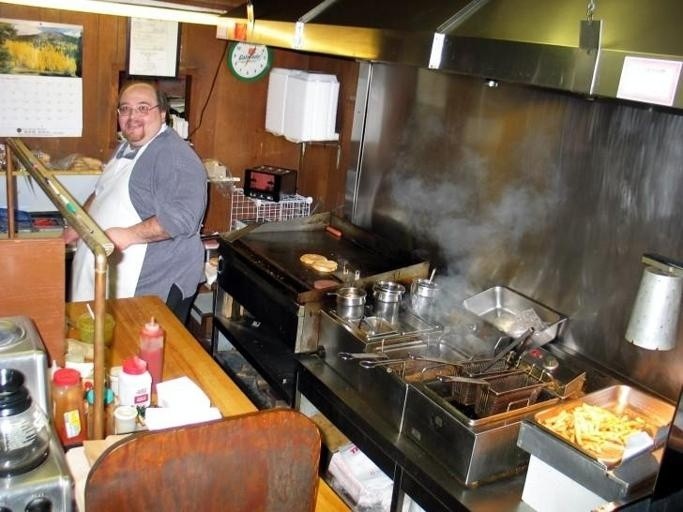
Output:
top-left (542, 402), bottom-right (645, 453)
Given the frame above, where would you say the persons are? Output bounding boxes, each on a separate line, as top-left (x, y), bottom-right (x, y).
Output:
top-left (63, 79), bottom-right (208, 324)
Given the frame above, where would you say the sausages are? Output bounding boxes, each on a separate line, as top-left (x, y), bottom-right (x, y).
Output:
top-left (326, 226), bottom-right (342, 237)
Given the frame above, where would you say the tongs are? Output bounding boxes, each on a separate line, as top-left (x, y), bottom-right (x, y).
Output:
top-left (475, 326), bottom-right (535, 374)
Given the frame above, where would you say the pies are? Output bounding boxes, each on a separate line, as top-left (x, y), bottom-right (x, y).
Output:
top-left (300, 254), bottom-right (337, 273)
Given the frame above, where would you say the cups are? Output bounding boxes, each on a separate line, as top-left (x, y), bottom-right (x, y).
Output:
top-left (76, 310), bottom-right (115, 344)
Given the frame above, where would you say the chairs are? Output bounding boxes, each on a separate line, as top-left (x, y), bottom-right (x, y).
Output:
top-left (84, 405), bottom-right (323, 512)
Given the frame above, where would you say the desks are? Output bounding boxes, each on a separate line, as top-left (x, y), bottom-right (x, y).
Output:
top-left (68, 294), bottom-right (352, 511)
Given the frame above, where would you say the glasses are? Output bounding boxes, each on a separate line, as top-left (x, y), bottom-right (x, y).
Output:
top-left (117, 103), bottom-right (157, 115)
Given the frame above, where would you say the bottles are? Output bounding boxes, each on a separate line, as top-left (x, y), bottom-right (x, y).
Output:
top-left (118, 316), bottom-right (165, 433)
top-left (335, 279), bottom-right (442, 320)
top-left (1, 367), bottom-right (52, 474)
top-left (52, 369), bottom-right (116, 448)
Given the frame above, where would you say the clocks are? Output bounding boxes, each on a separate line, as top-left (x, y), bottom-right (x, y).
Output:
top-left (228, 42), bottom-right (274, 84)
